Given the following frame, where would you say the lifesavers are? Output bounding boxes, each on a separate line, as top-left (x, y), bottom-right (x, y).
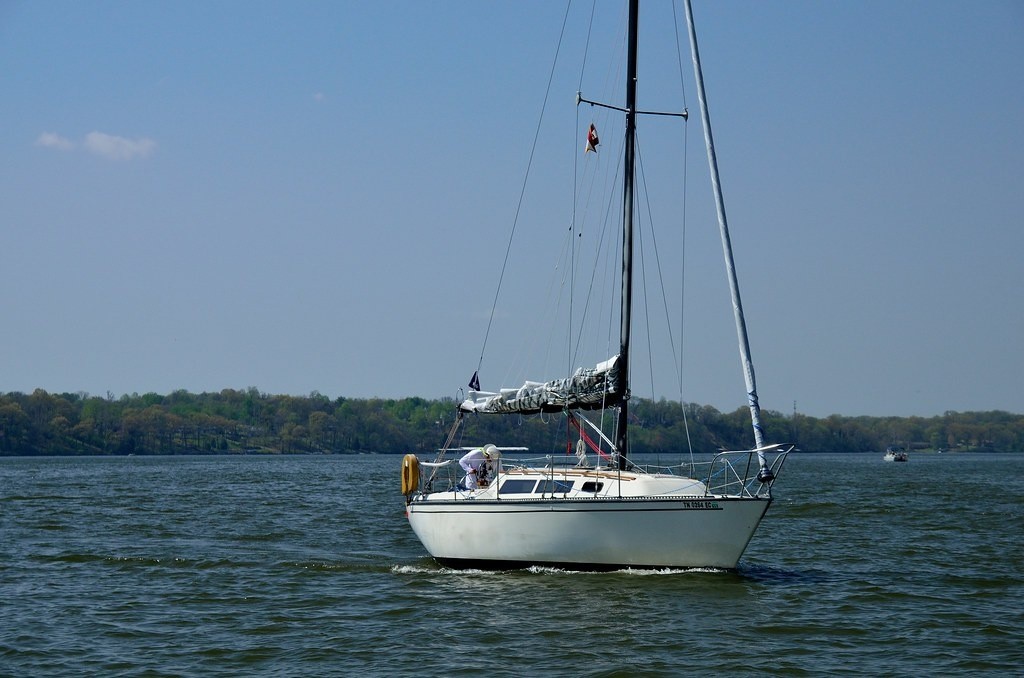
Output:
top-left (401, 451), bottom-right (421, 497)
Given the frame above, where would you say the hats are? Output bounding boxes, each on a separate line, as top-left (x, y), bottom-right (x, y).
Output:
top-left (487, 446), bottom-right (500, 460)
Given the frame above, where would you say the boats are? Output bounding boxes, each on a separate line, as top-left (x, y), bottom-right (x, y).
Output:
top-left (884, 444), bottom-right (910, 462)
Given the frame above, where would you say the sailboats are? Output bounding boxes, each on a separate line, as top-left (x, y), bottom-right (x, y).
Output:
top-left (400, 0), bottom-right (798, 571)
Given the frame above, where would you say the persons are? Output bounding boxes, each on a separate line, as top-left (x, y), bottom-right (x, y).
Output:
top-left (459, 444), bottom-right (503, 490)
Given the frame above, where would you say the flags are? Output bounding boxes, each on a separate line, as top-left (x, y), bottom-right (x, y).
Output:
top-left (584, 123), bottom-right (600, 155)
